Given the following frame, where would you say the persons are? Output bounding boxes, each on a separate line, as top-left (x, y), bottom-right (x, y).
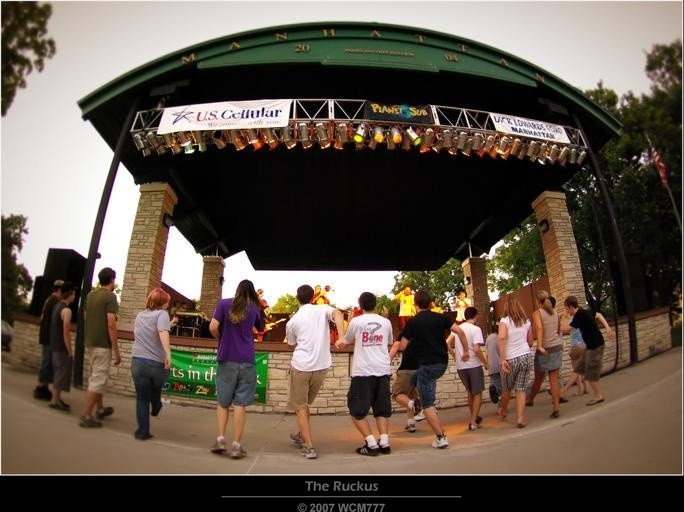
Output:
top-left (524, 289), bottom-right (564, 419)
top-left (48, 283), bottom-right (75, 412)
top-left (496, 294), bottom-right (534, 428)
top-left (559, 296), bottom-right (613, 406)
top-left (310, 285), bottom-right (330, 306)
top-left (78, 267), bottom-right (122, 428)
top-left (392, 317), bottom-right (421, 433)
top-left (397, 290), bottom-right (471, 450)
top-left (284, 285), bottom-right (345, 459)
top-left (33, 280), bottom-right (65, 402)
top-left (451, 288), bottom-right (471, 328)
top-left (485, 325), bottom-right (502, 405)
top-left (207, 280), bottom-right (267, 458)
top-left (256, 288), bottom-right (267, 311)
top-left (446, 306), bottom-right (490, 431)
top-left (559, 328), bottom-right (590, 403)
top-left (130, 288), bottom-right (178, 441)
top-left (391, 284), bottom-right (417, 329)
top-left (334, 292), bottom-right (394, 457)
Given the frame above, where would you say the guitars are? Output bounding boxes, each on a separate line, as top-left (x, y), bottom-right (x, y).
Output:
top-left (253, 318), bottom-right (286, 334)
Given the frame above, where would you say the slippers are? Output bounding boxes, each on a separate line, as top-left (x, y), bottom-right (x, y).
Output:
top-left (586, 397), bottom-right (604, 405)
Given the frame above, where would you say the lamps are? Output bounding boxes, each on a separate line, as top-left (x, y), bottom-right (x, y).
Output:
top-left (386, 135), bottom-right (396, 150)
top-left (248, 130), bottom-right (263, 151)
top-left (334, 127), bottom-right (345, 150)
top-left (316, 124), bottom-right (331, 150)
top-left (539, 219), bottom-right (549, 234)
top-left (213, 132), bottom-right (226, 150)
top-left (300, 124), bottom-right (313, 149)
top-left (432, 139), bottom-right (445, 154)
top-left (147, 132), bottom-right (165, 157)
top-left (461, 139), bottom-right (473, 157)
top-left (166, 139), bottom-right (183, 155)
top-left (281, 127), bottom-right (297, 150)
top-left (232, 130), bottom-right (247, 151)
top-left (577, 152), bottom-right (586, 165)
top-left (264, 128), bottom-right (281, 151)
top-left (132, 135), bottom-right (150, 157)
top-left (569, 149), bottom-right (577, 164)
top-left (484, 136), bottom-right (495, 154)
top-left (547, 144), bottom-right (557, 160)
top-left (401, 133), bottom-right (410, 151)
top-left (526, 140), bottom-right (537, 158)
top-left (406, 127), bottom-right (423, 147)
top-left (558, 148), bottom-right (569, 162)
top-left (471, 134), bottom-right (482, 152)
top-left (449, 136), bottom-right (458, 156)
top-left (391, 126), bottom-right (402, 144)
top-left (373, 125), bottom-right (385, 143)
top-left (497, 136), bottom-right (507, 155)
top-left (179, 134), bottom-right (195, 154)
top-left (511, 139), bottom-right (520, 157)
top-left (353, 124), bottom-right (366, 143)
top-left (164, 213), bottom-right (173, 226)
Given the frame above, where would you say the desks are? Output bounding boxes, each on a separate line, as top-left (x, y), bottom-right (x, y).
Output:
top-left (175, 312), bottom-right (204, 338)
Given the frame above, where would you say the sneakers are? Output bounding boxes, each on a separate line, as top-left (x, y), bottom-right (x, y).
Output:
top-left (299, 443), bottom-right (317, 458)
top-left (550, 410), bottom-right (560, 418)
top-left (525, 402), bottom-right (534, 406)
top-left (414, 406), bottom-right (438, 421)
top-left (96, 407), bottom-right (114, 419)
top-left (403, 423), bottom-right (416, 432)
top-left (79, 415), bottom-right (102, 428)
top-left (477, 415), bottom-right (482, 423)
top-left (210, 438), bottom-right (226, 453)
top-left (377, 438), bottom-right (391, 454)
top-left (469, 423), bottom-right (480, 431)
top-left (34, 387), bottom-right (51, 400)
top-left (412, 398), bottom-right (419, 415)
top-left (356, 440), bottom-right (379, 456)
top-left (231, 445), bottom-right (247, 458)
top-left (432, 435), bottom-right (448, 448)
top-left (50, 400), bottom-right (69, 410)
top-left (288, 431), bottom-right (304, 446)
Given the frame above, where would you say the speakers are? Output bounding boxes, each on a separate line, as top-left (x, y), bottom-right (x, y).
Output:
top-left (31, 248), bottom-right (86, 322)
